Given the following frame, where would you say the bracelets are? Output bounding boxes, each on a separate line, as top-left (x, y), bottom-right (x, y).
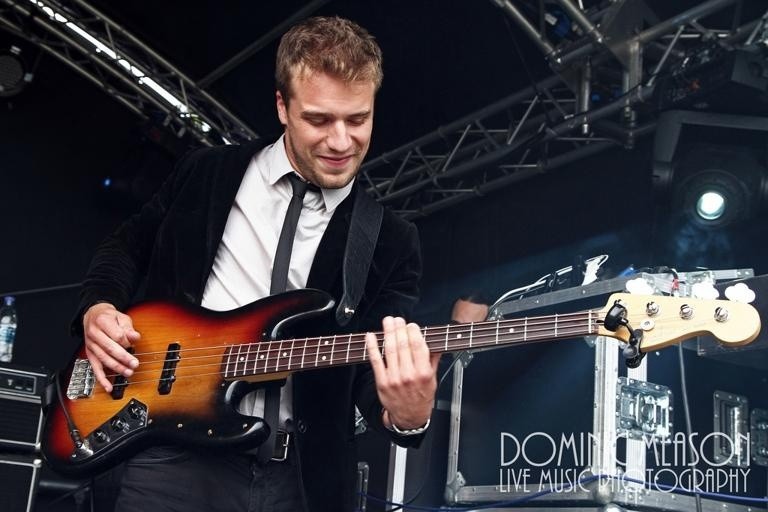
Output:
top-left (388, 410), bottom-right (430, 435)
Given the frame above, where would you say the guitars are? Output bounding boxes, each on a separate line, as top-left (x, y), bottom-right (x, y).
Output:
top-left (40, 277), bottom-right (761, 476)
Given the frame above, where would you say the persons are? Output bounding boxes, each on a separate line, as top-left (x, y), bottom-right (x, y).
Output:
top-left (70, 16), bottom-right (443, 512)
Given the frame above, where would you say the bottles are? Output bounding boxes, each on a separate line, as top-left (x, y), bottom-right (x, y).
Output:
top-left (0, 298), bottom-right (20, 365)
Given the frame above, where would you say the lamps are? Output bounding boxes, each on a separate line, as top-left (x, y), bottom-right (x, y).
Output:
top-left (673, 159), bottom-right (750, 235)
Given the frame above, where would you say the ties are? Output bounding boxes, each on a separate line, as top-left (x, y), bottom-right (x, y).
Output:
top-left (257, 172), bottom-right (320, 466)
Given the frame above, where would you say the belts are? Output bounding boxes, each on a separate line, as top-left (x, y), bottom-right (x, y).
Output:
top-left (270, 428), bottom-right (295, 468)
